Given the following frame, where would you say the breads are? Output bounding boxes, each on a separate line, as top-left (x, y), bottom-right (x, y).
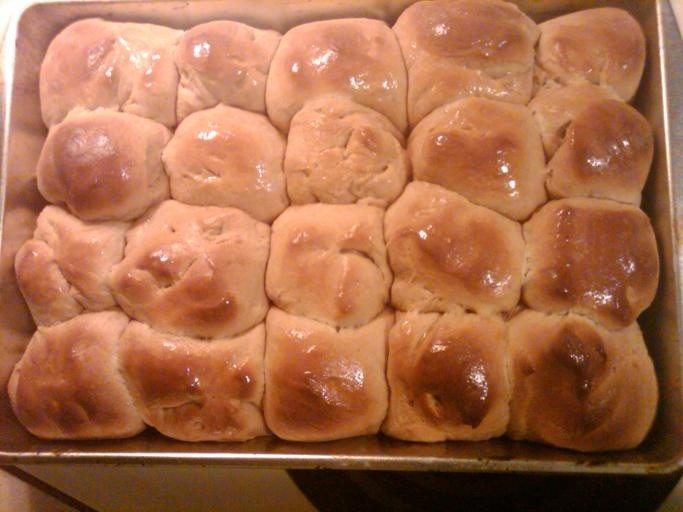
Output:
top-left (6, 2), bottom-right (669, 452)
